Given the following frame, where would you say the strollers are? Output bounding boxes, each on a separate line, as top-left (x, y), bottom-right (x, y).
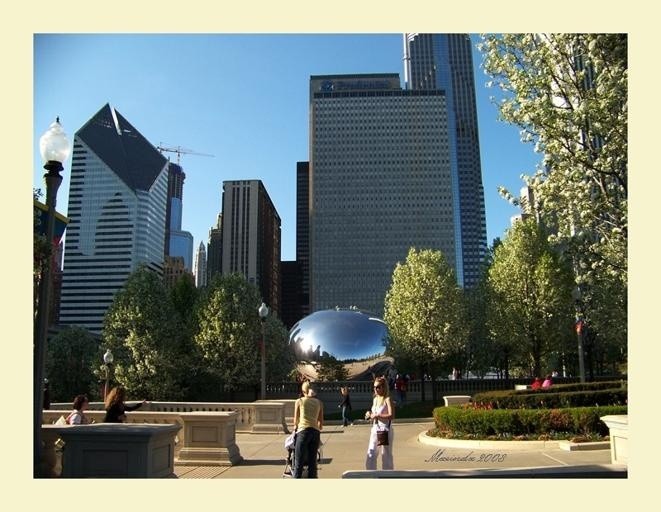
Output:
top-left (282, 425), bottom-right (324, 478)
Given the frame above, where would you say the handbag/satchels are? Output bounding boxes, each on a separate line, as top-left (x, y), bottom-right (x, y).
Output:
top-left (54, 413), bottom-right (81, 425)
top-left (376, 430), bottom-right (389, 446)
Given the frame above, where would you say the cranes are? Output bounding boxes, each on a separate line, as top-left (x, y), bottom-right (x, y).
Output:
top-left (156, 142), bottom-right (217, 166)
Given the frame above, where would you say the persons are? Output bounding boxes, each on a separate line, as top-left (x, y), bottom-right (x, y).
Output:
top-left (552, 369), bottom-right (560, 377)
top-left (365, 376), bottom-right (395, 470)
top-left (103, 385), bottom-right (148, 423)
top-left (338, 385), bottom-right (354, 427)
top-left (43, 378), bottom-right (50, 410)
top-left (396, 372), bottom-right (416, 408)
top-left (292, 380), bottom-right (324, 479)
top-left (66, 394), bottom-right (90, 425)
top-left (541, 376), bottom-right (553, 389)
top-left (532, 376), bottom-right (543, 389)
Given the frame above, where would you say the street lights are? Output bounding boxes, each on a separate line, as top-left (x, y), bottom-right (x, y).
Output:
top-left (103, 349), bottom-right (115, 400)
top-left (257, 303), bottom-right (268, 401)
top-left (571, 285), bottom-right (586, 383)
top-left (35, 115), bottom-right (70, 478)
